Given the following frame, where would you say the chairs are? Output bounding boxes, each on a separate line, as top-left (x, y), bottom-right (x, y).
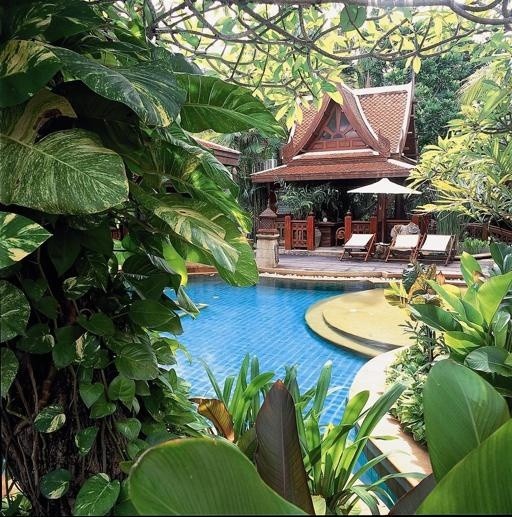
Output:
top-left (339, 233), bottom-right (376, 262)
top-left (414, 232), bottom-right (457, 265)
top-left (383, 233), bottom-right (423, 263)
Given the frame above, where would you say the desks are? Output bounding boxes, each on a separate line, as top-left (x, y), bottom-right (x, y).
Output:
top-left (373, 242), bottom-right (390, 258)
